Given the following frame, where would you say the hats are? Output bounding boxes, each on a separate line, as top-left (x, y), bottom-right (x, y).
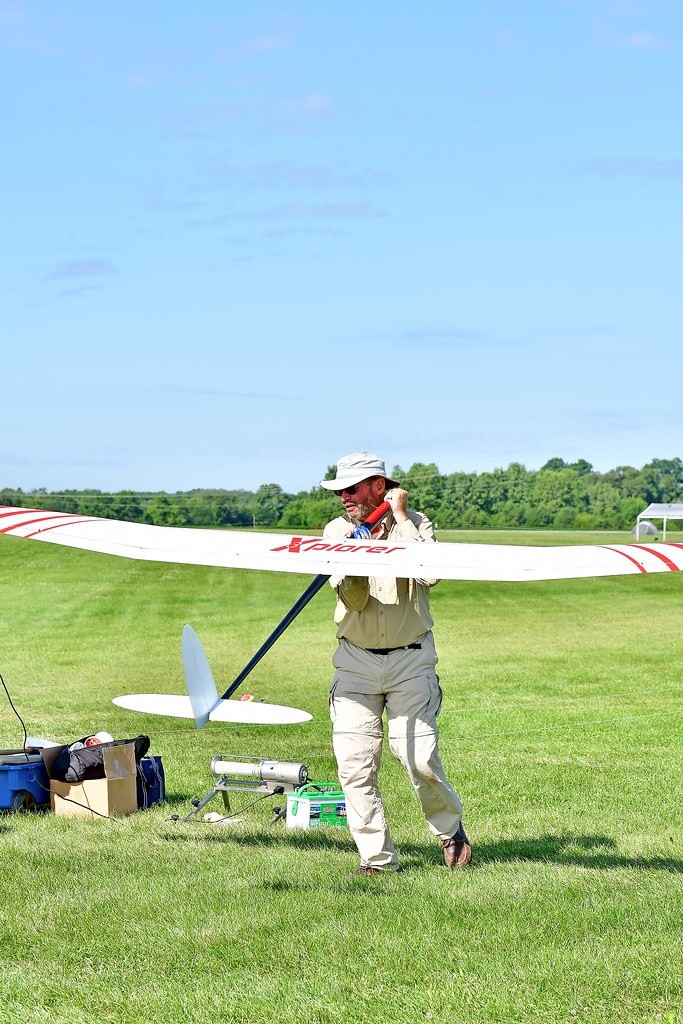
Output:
top-left (320, 452), bottom-right (401, 490)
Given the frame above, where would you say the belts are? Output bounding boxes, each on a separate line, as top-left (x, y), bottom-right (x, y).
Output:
top-left (366, 643), bottom-right (421, 655)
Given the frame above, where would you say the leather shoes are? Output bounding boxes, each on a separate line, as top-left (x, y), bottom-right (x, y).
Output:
top-left (350, 866), bottom-right (398, 876)
top-left (442, 821), bottom-right (472, 869)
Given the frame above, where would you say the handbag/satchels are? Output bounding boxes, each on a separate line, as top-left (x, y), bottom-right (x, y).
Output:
top-left (52, 734), bottom-right (150, 782)
top-left (137, 757), bottom-right (165, 811)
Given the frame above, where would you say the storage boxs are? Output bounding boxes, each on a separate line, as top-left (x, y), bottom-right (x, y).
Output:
top-left (40, 742), bottom-right (137, 822)
top-left (284, 782), bottom-right (346, 832)
top-left (0, 754), bottom-right (51, 813)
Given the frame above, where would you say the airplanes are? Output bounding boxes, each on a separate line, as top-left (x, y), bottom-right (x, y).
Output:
top-left (0, 505), bottom-right (683, 725)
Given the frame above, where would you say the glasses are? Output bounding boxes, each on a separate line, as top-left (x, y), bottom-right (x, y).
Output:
top-left (333, 478), bottom-right (375, 496)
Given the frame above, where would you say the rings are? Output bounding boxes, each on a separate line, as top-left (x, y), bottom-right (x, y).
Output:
top-left (391, 490), bottom-right (393, 492)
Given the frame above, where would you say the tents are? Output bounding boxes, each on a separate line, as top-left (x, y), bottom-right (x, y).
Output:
top-left (632, 521), bottom-right (658, 535)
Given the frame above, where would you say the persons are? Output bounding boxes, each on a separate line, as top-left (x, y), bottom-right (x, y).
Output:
top-left (322, 452), bottom-right (471, 877)
